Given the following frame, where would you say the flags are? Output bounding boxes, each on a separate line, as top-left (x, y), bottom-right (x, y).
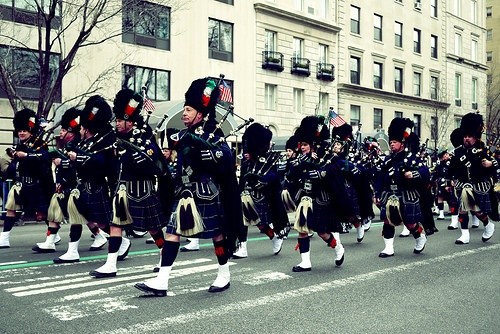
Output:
top-left (219, 80), bottom-right (232, 102)
top-left (142, 94), bottom-right (155, 114)
top-left (328, 110), bottom-right (345, 127)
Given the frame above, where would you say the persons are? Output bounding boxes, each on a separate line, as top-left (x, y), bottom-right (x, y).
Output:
top-left (0, 89), bottom-right (500, 278)
top-left (135, 77), bottom-right (236, 297)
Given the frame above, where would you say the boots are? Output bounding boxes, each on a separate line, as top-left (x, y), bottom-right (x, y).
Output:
top-left (54, 233), bottom-right (61, 244)
top-left (186, 238), bottom-right (193, 241)
top-left (117, 237), bottom-right (131, 259)
top-left (208, 240), bottom-right (230, 292)
top-left (0, 230), bottom-right (11, 248)
top-left (90, 236), bottom-right (122, 277)
top-left (32, 227), bottom-right (58, 251)
top-left (180, 236), bottom-right (200, 250)
top-left (89, 226), bottom-right (107, 250)
top-left (233, 203), bottom-right (496, 273)
top-left (53, 223), bottom-right (83, 264)
top-left (134, 240), bottom-right (180, 296)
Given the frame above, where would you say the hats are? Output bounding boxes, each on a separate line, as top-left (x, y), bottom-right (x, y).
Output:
top-left (113, 89), bottom-right (142, 123)
top-left (13, 109), bottom-right (36, 135)
top-left (332, 123), bottom-right (352, 142)
top-left (460, 114), bottom-right (484, 139)
top-left (387, 118), bottom-right (414, 144)
top-left (61, 109), bottom-right (82, 133)
top-left (241, 123), bottom-right (272, 156)
top-left (80, 96), bottom-right (111, 134)
top-left (297, 116), bottom-right (329, 148)
top-left (184, 78), bottom-right (220, 116)
top-left (285, 136), bottom-right (298, 153)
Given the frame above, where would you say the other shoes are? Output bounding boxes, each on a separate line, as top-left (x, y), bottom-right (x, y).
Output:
top-left (146, 238), bottom-right (155, 243)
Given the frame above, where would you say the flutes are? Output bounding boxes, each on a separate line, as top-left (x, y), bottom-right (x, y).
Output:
top-left (18, 72), bottom-right (453, 189)
